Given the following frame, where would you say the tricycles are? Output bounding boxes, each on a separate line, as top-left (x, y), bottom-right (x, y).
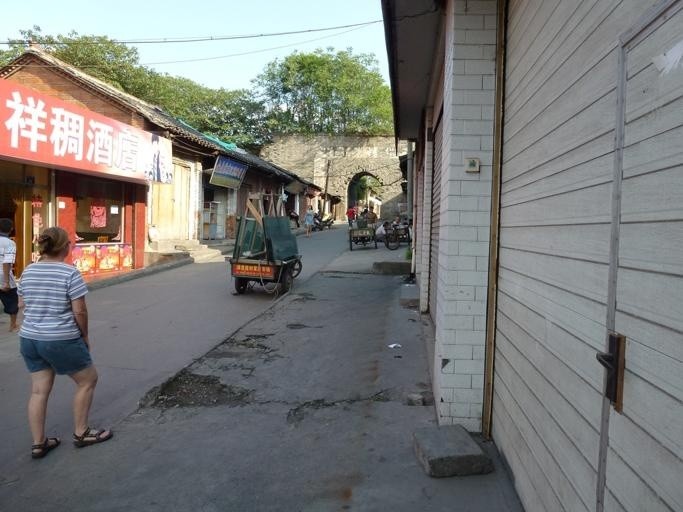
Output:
top-left (347, 218), bottom-right (378, 251)
top-left (384, 224), bottom-right (411, 250)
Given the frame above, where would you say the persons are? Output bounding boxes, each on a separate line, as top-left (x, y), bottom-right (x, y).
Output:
top-left (14, 227), bottom-right (113, 459)
top-left (289, 208), bottom-right (300, 227)
top-left (376, 221), bottom-right (389, 237)
top-left (0, 218), bottom-right (19, 332)
top-left (365, 206), bottom-right (377, 224)
top-left (393, 216), bottom-right (402, 224)
top-left (364, 206), bottom-right (369, 214)
top-left (304, 205), bottom-right (314, 238)
top-left (346, 206), bottom-right (354, 229)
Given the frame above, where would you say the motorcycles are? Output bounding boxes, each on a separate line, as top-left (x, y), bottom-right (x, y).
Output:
top-left (229, 255), bottom-right (303, 296)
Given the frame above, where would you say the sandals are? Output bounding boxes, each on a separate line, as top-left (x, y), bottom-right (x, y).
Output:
top-left (32, 437), bottom-right (61, 457)
top-left (73, 427), bottom-right (113, 447)
